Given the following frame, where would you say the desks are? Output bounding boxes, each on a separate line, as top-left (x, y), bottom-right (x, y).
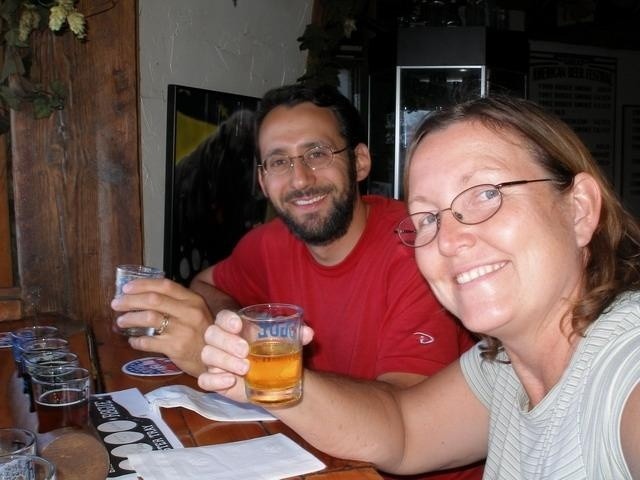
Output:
top-left (0, 318), bottom-right (384, 480)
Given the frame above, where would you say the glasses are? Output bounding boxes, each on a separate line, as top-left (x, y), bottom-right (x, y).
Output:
top-left (255, 145), bottom-right (349, 176)
top-left (392, 178), bottom-right (554, 248)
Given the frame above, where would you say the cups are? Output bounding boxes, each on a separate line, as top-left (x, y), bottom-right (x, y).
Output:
top-left (10, 323), bottom-right (91, 431)
top-left (110, 263), bottom-right (165, 337)
top-left (236, 305), bottom-right (308, 406)
top-left (0, 429), bottom-right (61, 480)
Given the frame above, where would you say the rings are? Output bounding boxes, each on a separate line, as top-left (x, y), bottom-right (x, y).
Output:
top-left (155, 312), bottom-right (170, 334)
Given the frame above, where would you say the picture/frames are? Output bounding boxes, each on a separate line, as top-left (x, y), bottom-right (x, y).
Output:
top-left (393, 65), bottom-right (486, 198)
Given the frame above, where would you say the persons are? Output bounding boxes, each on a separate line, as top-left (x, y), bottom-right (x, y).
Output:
top-left (197, 91), bottom-right (640, 480)
top-left (109, 82), bottom-right (477, 388)
